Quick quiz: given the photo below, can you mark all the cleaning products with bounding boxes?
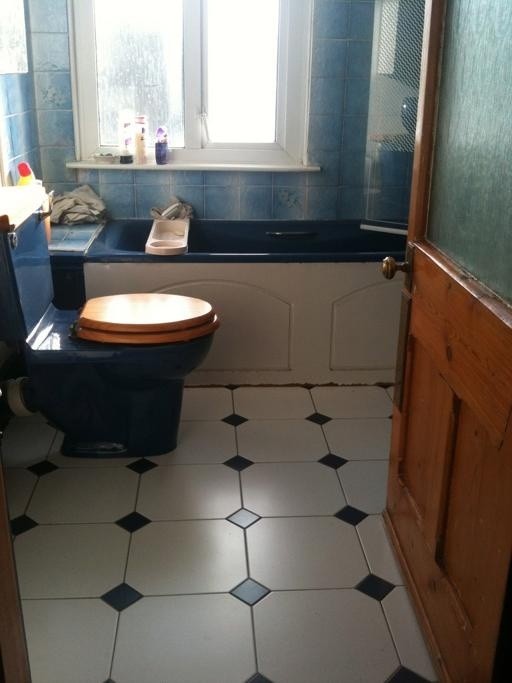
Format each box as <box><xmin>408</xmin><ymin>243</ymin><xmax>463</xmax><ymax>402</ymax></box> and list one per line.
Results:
<box><xmin>17</xmin><ymin>162</ymin><xmax>52</xmax><ymax>246</ymax></box>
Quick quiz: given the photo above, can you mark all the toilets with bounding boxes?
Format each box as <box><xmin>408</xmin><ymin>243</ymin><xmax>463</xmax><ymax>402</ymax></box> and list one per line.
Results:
<box><xmin>1</xmin><ymin>180</ymin><xmax>218</xmax><ymax>458</ymax></box>
